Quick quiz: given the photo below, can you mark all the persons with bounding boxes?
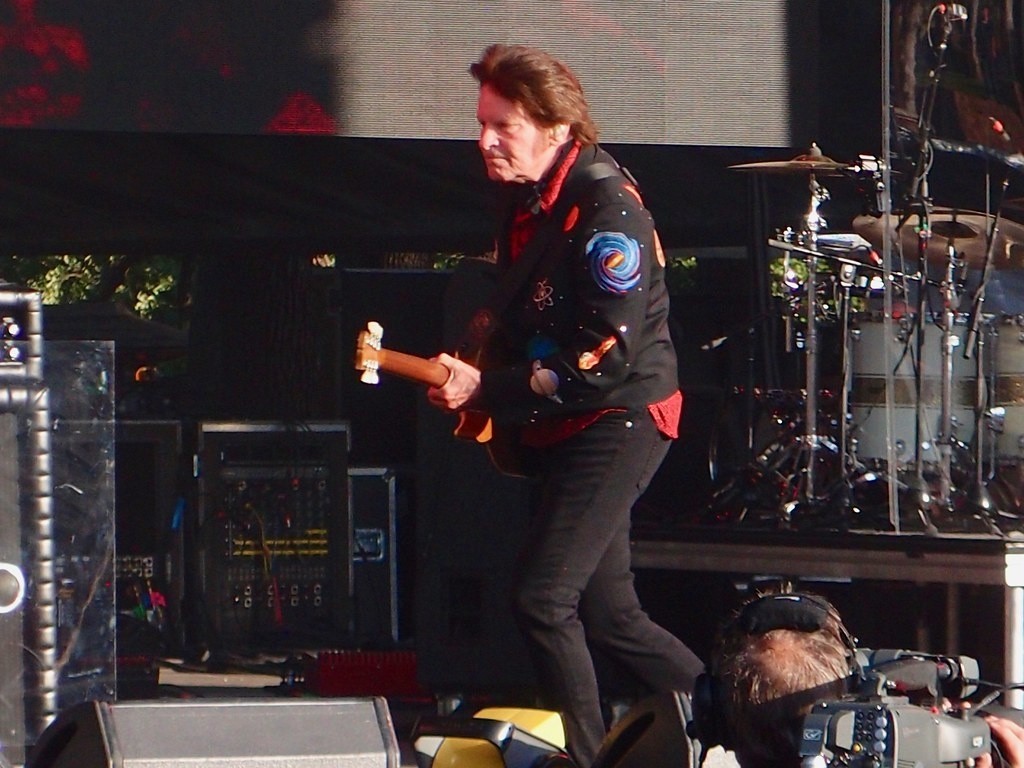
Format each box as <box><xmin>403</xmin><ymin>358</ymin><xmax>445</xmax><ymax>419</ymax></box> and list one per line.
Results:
<box><xmin>710</xmin><ymin>581</ymin><xmax>1024</xmax><ymax>768</ymax></box>
<box><xmin>427</xmin><ymin>43</ymin><xmax>708</xmax><ymax>768</ymax></box>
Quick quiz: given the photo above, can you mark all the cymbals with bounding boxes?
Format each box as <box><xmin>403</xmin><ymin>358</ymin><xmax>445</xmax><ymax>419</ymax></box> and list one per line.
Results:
<box><xmin>732</xmin><ymin>141</ymin><xmax>857</xmax><ymax>171</ymax></box>
<box><xmin>853</xmin><ymin>206</ymin><xmax>1024</xmax><ymax>270</ymax></box>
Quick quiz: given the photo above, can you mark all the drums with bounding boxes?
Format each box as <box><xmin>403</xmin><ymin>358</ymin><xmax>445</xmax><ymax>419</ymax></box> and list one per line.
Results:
<box><xmin>845</xmin><ymin>255</ymin><xmax>1024</xmax><ymax>475</ymax></box>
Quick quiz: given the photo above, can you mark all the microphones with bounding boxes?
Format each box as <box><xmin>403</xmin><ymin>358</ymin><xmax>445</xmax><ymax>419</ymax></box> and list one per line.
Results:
<box><xmin>937</xmin><ymin>4</ymin><xmax>967</xmax><ymax>19</ymax></box>
<box><xmin>989</xmin><ymin>115</ymin><xmax>1011</xmax><ymax>141</ymax></box>
<box><xmin>865</xmin><ymin>246</ymin><xmax>903</xmax><ymax>296</ymax></box>
<box><xmin>842</xmin><ymin>167</ymin><xmax>897</xmax><ymax>188</ymax></box>
<box><xmin>936</xmin><ymin>656</ymin><xmax>979</xmax><ymax>698</ymax></box>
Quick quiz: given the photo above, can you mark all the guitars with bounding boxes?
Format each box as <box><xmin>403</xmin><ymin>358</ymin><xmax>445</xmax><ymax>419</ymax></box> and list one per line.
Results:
<box><xmin>354</xmin><ymin>307</ymin><xmax>535</xmax><ymax>479</ymax></box>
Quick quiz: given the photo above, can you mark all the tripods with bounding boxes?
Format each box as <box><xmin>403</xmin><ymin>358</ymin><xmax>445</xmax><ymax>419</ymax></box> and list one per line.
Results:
<box><xmin>689</xmin><ymin>25</ymin><xmax>1024</xmax><ymax>541</ymax></box>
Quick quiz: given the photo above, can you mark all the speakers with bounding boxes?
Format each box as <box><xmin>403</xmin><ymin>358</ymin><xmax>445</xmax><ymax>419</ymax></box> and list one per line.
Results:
<box><xmin>25</xmin><ymin>695</ymin><xmax>400</xmax><ymax>768</ymax></box>
<box><xmin>337</xmin><ymin>266</ymin><xmax>559</xmax><ymax>697</ymax></box>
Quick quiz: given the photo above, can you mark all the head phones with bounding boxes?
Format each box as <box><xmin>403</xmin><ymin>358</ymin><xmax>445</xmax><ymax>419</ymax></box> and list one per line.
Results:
<box><xmin>686</xmin><ymin>594</ymin><xmax>871</xmax><ymax>750</ymax></box>
